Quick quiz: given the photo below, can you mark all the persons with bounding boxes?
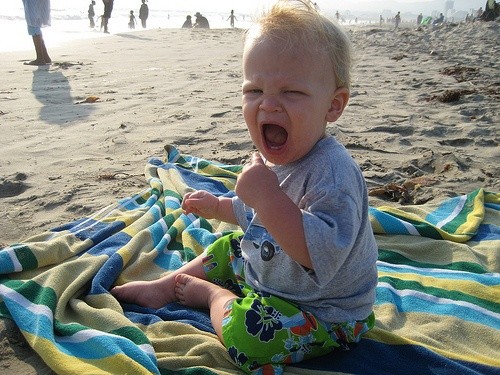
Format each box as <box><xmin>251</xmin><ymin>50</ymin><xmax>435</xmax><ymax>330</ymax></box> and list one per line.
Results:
<box><xmin>22</xmin><ymin>0</ymin><xmax>51</xmax><ymax>66</ymax></box>
<box><xmin>89</xmin><ymin>0</ymin><xmax>500</xmax><ymax>33</ymax></box>
<box><xmin>110</xmin><ymin>0</ymin><xmax>379</xmax><ymax>375</ymax></box>
